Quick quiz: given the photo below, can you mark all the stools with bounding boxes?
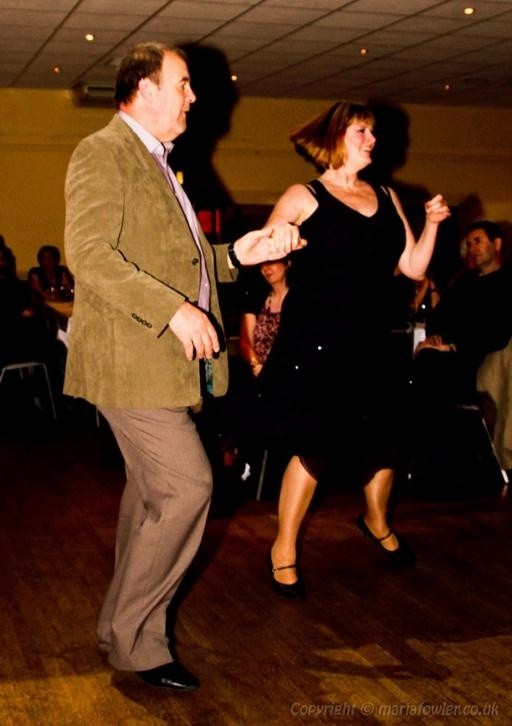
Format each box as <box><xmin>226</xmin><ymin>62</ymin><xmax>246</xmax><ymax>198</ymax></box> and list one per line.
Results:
<box><xmin>0</xmin><ymin>360</ymin><xmax>57</xmax><ymax>420</ymax></box>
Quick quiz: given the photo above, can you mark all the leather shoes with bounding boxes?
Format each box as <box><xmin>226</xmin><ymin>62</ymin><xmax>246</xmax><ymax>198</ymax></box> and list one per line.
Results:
<box><xmin>265</xmin><ymin>549</ymin><xmax>303</xmax><ymax>599</ymax></box>
<box><xmin>138</xmin><ymin>663</ymin><xmax>199</xmax><ymax>692</ymax></box>
<box><xmin>358</xmin><ymin>518</ymin><xmax>409</xmax><ymax>562</ymax></box>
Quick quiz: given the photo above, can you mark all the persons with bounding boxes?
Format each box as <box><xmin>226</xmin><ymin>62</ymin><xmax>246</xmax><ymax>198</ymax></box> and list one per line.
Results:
<box><xmin>402</xmin><ymin>220</ymin><xmax>512</xmax><ymax>405</ymax></box>
<box><xmin>244</xmin><ymin>255</ymin><xmax>290</xmax><ymax>376</ymax></box>
<box><xmin>62</xmin><ymin>41</ymin><xmax>291</xmax><ymax>694</ymax></box>
<box><xmin>26</xmin><ymin>244</ymin><xmax>73</xmax><ymax>301</ymax></box>
<box><xmin>0</xmin><ymin>235</ymin><xmax>66</xmax><ymax>396</ymax></box>
<box><xmin>261</xmin><ymin>98</ymin><xmax>451</xmax><ymax>601</ymax></box>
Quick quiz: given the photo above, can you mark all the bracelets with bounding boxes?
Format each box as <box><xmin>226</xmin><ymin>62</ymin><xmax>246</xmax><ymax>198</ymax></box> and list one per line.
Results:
<box><xmin>228</xmin><ymin>242</ymin><xmax>241</xmax><ymax>269</ymax></box>
